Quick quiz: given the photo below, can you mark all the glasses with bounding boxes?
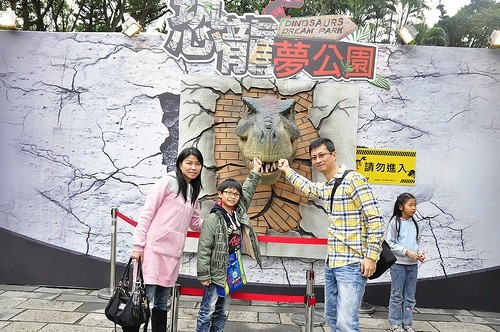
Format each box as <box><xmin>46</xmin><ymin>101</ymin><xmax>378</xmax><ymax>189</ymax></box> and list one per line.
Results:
<box><xmin>310</xmin><ymin>152</ymin><xmax>332</xmax><ymax>162</ymax></box>
<box><xmin>223</xmin><ymin>191</ymin><xmax>241</xmax><ymax>197</ymax></box>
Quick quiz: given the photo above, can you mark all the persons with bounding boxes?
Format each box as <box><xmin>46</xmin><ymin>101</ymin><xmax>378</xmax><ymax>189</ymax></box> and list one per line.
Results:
<box><xmin>278</xmin><ymin>138</ymin><xmax>384</xmax><ymax>332</ymax></box>
<box><xmin>125</xmin><ymin>148</ymin><xmax>204</xmax><ymax>332</ymax></box>
<box><xmin>387</xmin><ymin>193</ymin><xmax>426</xmax><ymax>332</ymax></box>
<box><xmin>195</xmin><ymin>156</ymin><xmax>262</xmax><ymax>332</ymax></box>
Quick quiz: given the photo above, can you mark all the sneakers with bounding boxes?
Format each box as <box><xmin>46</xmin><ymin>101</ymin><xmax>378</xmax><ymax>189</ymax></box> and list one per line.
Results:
<box><xmin>390</xmin><ymin>325</ymin><xmax>415</xmax><ymax>332</ymax></box>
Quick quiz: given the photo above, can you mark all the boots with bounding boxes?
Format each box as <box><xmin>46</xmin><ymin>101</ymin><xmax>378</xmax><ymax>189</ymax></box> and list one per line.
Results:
<box><xmin>151</xmin><ymin>307</ymin><xmax>167</xmax><ymax>332</ymax></box>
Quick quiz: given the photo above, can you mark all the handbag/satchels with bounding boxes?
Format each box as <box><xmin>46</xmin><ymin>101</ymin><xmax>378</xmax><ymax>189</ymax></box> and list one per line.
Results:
<box><xmin>105</xmin><ymin>256</ymin><xmax>150</xmax><ymax>327</ymax></box>
<box><xmin>216</xmin><ymin>249</ymin><xmax>247</xmax><ymax>297</ymax></box>
<box><xmin>367</xmin><ymin>240</ymin><xmax>397</xmax><ymax>280</ymax></box>
